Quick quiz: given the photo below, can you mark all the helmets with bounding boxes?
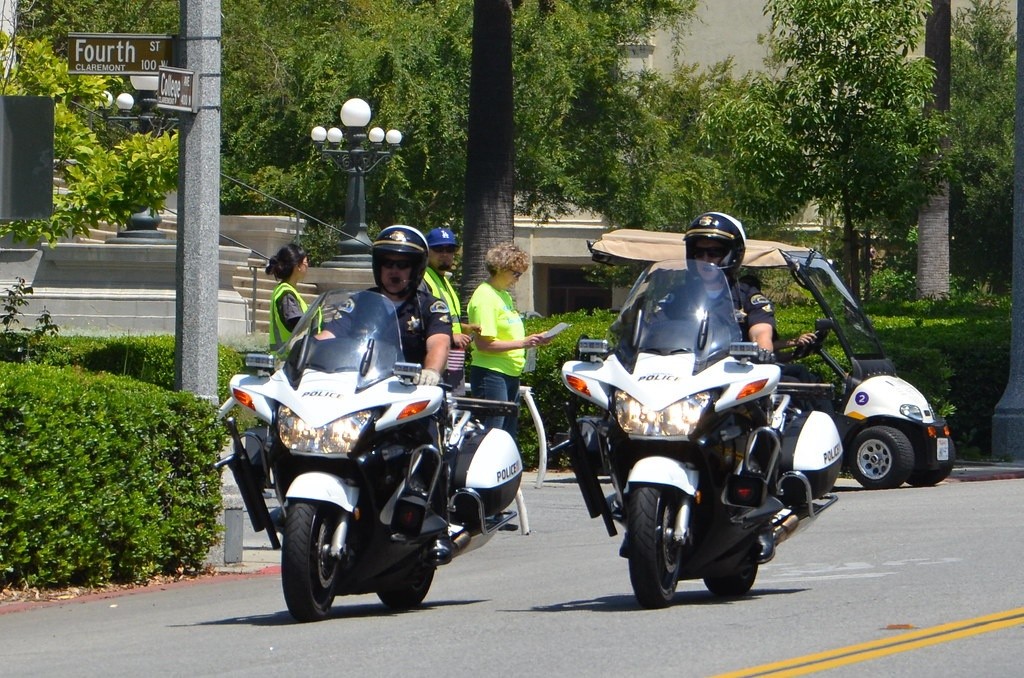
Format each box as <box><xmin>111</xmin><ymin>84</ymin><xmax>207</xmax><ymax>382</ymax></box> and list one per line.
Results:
<box><xmin>683</xmin><ymin>211</ymin><xmax>746</xmax><ymax>275</ymax></box>
<box><xmin>372</xmin><ymin>224</ymin><xmax>429</xmax><ymax>289</ymax></box>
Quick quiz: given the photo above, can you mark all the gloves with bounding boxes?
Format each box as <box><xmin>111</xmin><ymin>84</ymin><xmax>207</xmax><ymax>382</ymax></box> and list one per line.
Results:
<box><xmin>413</xmin><ymin>368</ymin><xmax>443</xmax><ymax>386</ymax></box>
<box><xmin>747</xmin><ymin>348</ymin><xmax>776</xmax><ymax>364</ymax></box>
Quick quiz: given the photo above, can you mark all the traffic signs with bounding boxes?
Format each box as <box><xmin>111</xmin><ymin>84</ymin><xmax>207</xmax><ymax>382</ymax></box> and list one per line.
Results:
<box><xmin>67</xmin><ymin>31</ymin><xmax>170</xmax><ymax>75</ymax></box>
<box><xmin>155</xmin><ymin>67</ymin><xmax>199</xmax><ymax>114</ymax></box>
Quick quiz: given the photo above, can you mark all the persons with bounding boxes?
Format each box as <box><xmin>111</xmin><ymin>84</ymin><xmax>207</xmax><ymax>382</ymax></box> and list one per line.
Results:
<box><xmin>467</xmin><ymin>241</ymin><xmax>555</xmax><ymax>531</ymax></box>
<box><xmin>650</xmin><ymin>212</ymin><xmax>772</xmax><ymax>471</ymax></box>
<box><xmin>316</xmin><ymin>225</ymin><xmax>453</xmax><ymax>564</ymax></box>
<box><xmin>738</xmin><ymin>275</ymin><xmax>836</xmax><ymax>419</ymax></box>
<box><xmin>417</xmin><ymin>227</ymin><xmax>481</xmax><ymax>398</ymax></box>
<box><xmin>265</xmin><ymin>243</ymin><xmax>308</xmax><ymax>360</ymax></box>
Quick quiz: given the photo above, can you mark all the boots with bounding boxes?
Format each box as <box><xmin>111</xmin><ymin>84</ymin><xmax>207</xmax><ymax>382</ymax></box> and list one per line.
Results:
<box><xmin>269</xmin><ymin>506</ymin><xmax>290</xmax><ymax>529</ymax></box>
<box><xmin>606</xmin><ymin>492</ymin><xmax>630</xmax><ymax>520</ymax></box>
<box><xmin>741</xmin><ymin>429</ymin><xmax>783</xmax><ymax>564</ymax></box>
<box><xmin>425</xmin><ymin>461</ymin><xmax>452</xmax><ymax>565</ymax></box>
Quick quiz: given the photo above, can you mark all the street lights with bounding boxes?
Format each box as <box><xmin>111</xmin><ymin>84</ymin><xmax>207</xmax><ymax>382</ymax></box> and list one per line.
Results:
<box><xmin>93</xmin><ymin>75</ymin><xmax>179</xmax><ymax>246</ymax></box>
<box><xmin>310</xmin><ymin>97</ymin><xmax>403</xmax><ymax>266</ymax></box>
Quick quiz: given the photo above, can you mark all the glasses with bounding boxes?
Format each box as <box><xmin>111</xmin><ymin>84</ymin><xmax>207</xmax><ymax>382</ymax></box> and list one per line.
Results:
<box><xmin>509</xmin><ymin>269</ymin><xmax>522</xmax><ymax>278</ymax></box>
<box><xmin>381</xmin><ymin>258</ymin><xmax>414</xmax><ymax>270</ymax></box>
<box><xmin>431</xmin><ymin>245</ymin><xmax>455</xmax><ymax>253</ymax></box>
<box><xmin>694</xmin><ymin>246</ymin><xmax>726</xmax><ymax>257</ymax></box>
<box><xmin>301</xmin><ymin>262</ymin><xmax>308</xmax><ymax>269</ymax></box>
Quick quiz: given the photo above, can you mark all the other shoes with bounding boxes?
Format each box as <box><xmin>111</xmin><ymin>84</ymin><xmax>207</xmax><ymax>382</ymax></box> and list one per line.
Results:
<box><xmin>495</xmin><ymin>514</ymin><xmax>518</xmax><ymax>531</ymax></box>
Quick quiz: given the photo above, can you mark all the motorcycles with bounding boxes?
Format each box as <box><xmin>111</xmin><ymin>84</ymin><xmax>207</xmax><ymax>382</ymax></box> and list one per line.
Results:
<box><xmin>554</xmin><ymin>260</ymin><xmax>844</xmax><ymax>610</ymax></box>
<box><xmin>216</xmin><ymin>289</ymin><xmax>525</xmax><ymax>621</ymax></box>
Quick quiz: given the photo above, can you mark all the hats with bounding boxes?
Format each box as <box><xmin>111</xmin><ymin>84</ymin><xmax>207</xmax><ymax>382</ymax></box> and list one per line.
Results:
<box><xmin>425</xmin><ymin>227</ymin><xmax>457</xmax><ymax>247</ymax></box>
